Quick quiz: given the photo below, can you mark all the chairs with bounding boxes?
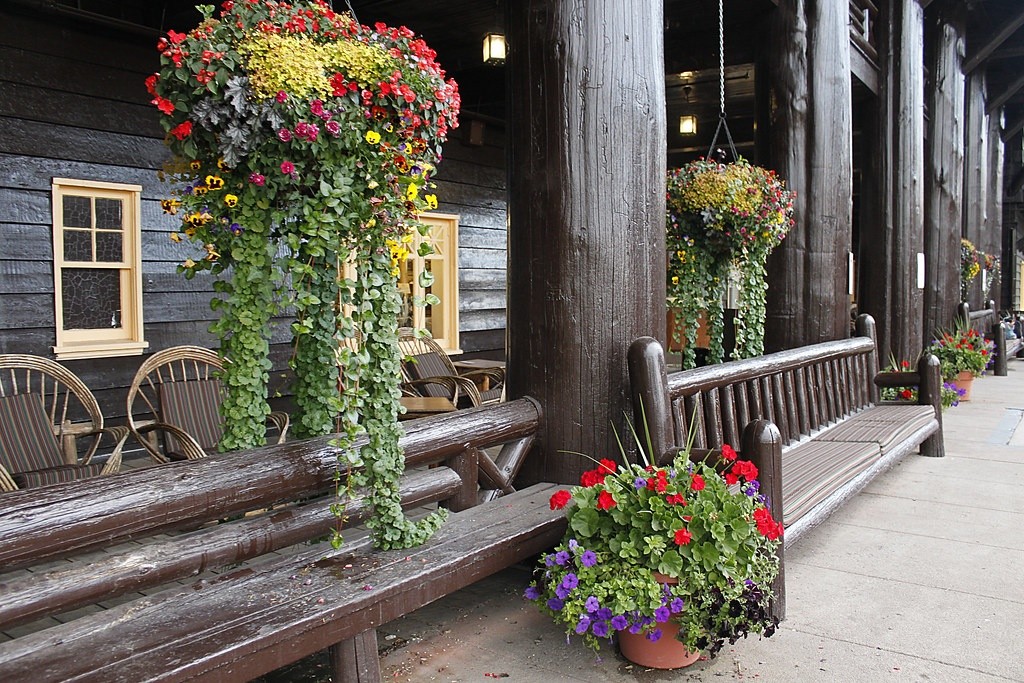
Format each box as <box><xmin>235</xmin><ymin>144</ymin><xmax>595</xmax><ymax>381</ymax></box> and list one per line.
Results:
<box><xmin>0</xmin><ymin>353</ymin><xmax>131</xmax><ymax>494</ymax></box>
<box><xmin>127</xmin><ymin>345</ymin><xmax>302</xmax><ymax>526</ymax></box>
<box><xmin>396</xmin><ymin>327</ymin><xmax>507</xmax><ymax>407</ymax></box>
<box><xmin>337</xmin><ymin>324</ymin><xmax>460</xmax><ymax>423</ymax></box>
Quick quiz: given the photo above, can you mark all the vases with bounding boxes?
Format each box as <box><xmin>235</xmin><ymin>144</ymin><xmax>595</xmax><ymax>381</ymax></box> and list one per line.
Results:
<box><xmin>615</xmin><ymin>571</ymin><xmax>701</xmax><ymax>669</ymax></box>
<box><xmin>946</xmin><ymin>369</ymin><xmax>974</xmax><ymax>402</ymax></box>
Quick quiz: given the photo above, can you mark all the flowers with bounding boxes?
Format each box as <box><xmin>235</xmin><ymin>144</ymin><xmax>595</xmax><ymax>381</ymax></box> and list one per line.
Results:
<box><xmin>667</xmin><ymin>148</ymin><xmax>800</xmax><ymax>381</ymax></box>
<box><xmin>143</xmin><ymin>0</ymin><xmax>452</xmax><ymax>558</ymax></box>
<box><xmin>879</xmin><ymin>347</ymin><xmax>966</xmax><ymax>412</ymax></box>
<box><xmin>982</xmin><ymin>253</ymin><xmax>1003</xmax><ymax>296</ymax></box>
<box><xmin>960</xmin><ymin>238</ymin><xmax>984</xmax><ymax>300</ymax></box>
<box><xmin>929</xmin><ymin>316</ymin><xmax>998</xmax><ymax>380</ymax></box>
<box><xmin>519</xmin><ymin>392</ymin><xmax>784</xmax><ymax>660</ymax></box>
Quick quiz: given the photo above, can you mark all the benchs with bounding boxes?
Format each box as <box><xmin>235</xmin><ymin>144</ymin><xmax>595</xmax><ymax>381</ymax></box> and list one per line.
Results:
<box><xmin>958</xmin><ymin>300</ymin><xmax>1024</xmax><ymax>377</ymax></box>
<box><xmin>0</xmin><ymin>394</ymin><xmax>585</xmax><ymax>683</ymax></box>
<box><xmin>625</xmin><ymin>313</ymin><xmax>947</xmax><ymax>623</ymax></box>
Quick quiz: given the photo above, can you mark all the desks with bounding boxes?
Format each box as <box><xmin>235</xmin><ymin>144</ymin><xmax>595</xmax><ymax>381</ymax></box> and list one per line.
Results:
<box><xmin>452</xmin><ymin>358</ymin><xmax>506</xmax><ymax>390</ymax></box>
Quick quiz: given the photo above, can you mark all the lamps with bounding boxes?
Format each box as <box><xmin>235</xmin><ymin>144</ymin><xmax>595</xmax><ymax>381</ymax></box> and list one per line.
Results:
<box><xmin>481</xmin><ymin>32</ymin><xmax>506</xmax><ymax>65</ymax></box>
<box><xmin>677</xmin><ymin>85</ymin><xmax>700</xmax><ymax>138</ymax></box>
